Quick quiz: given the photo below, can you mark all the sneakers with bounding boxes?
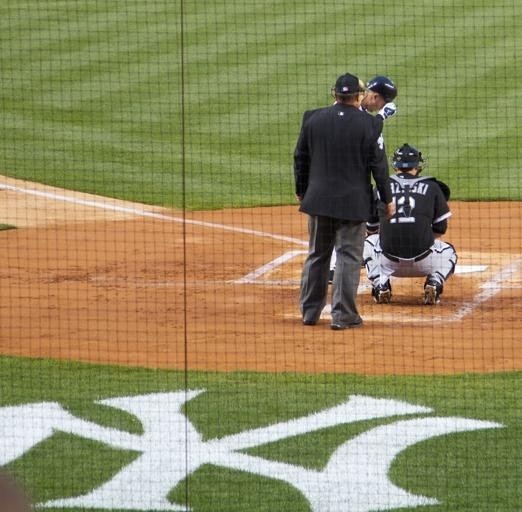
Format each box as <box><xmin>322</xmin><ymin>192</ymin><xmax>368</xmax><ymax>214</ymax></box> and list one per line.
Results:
<box><xmin>304</xmin><ymin>319</ymin><xmax>317</xmax><ymax>325</ymax></box>
<box><xmin>424</xmin><ymin>279</ymin><xmax>440</xmax><ymax>305</ymax></box>
<box><xmin>375</xmin><ymin>283</ymin><xmax>391</xmax><ymax>304</ymax></box>
<box><xmin>331</xmin><ymin>320</ymin><xmax>362</xmax><ymax>330</ymax></box>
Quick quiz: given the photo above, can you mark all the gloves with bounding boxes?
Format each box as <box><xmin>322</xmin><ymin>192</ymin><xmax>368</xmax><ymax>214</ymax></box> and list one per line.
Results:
<box><xmin>378</xmin><ymin>102</ymin><xmax>397</xmax><ymax>119</ymax></box>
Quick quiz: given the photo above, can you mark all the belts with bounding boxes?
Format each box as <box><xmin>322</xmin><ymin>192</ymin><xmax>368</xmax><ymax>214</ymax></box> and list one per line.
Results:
<box><xmin>381</xmin><ymin>251</ymin><xmax>432</xmax><ymax>262</ymax></box>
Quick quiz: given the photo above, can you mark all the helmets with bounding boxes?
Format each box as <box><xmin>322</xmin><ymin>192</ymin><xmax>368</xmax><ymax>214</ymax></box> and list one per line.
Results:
<box><xmin>392</xmin><ymin>144</ymin><xmax>423</xmax><ymax>175</ymax></box>
<box><xmin>331</xmin><ymin>73</ymin><xmax>365</xmax><ymax>96</ymax></box>
<box><xmin>366</xmin><ymin>76</ymin><xmax>397</xmax><ymax>102</ymax></box>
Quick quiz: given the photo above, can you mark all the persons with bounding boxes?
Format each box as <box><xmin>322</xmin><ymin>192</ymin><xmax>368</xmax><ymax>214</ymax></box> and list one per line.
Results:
<box><xmin>292</xmin><ymin>71</ymin><xmax>392</xmax><ymax>332</ymax></box>
<box><xmin>362</xmin><ymin>143</ymin><xmax>458</xmax><ymax>305</ymax></box>
<box><xmin>327</xmin><ymin>75</ymin><xmax>398</xmax><ymax>287</ymax></box>
